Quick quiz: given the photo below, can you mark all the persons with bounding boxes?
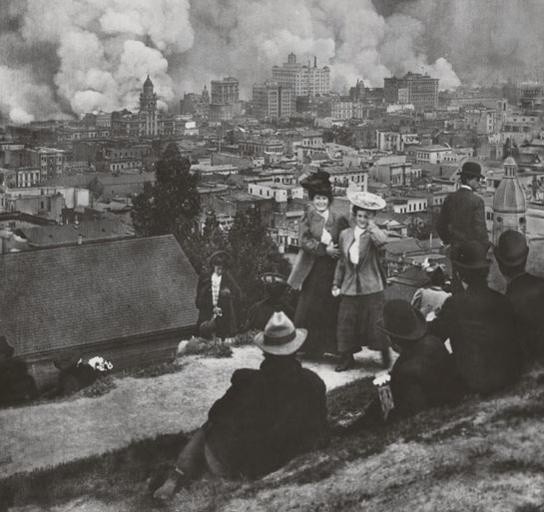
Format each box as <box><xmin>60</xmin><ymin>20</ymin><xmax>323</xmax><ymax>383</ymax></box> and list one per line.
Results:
<box><xmin>287</xmin><ymin>172</ymin><xmax>392</xmax><ymax>372</ymax></box>
<box><xmin>363</xmin><ymin>162</ymin><xmax>544</xmax><ymax>422</ymax></box>
<box><xmin>194</xmin><ymin>251</ymin><xmax>241</xmax><ymax>342</ymax></box>
<box><xmin>153</xmin><ymin>310</ymin><xmax>328</xmax><ymax>504</ymax></box>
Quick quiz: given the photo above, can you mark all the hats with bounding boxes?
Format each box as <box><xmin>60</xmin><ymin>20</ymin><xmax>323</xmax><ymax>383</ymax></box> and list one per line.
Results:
<box><xmin>376</xmin><ymin>299</ymin><xmax>428</xmax><ymax>341</ymax></box>
<box><xmin>495</xmin><ymin>229</ymin><xmax>526</xmax><ymax>268</ymax></box>
<box><xmin>346</xmin><ymin>191</ymin><xmax>386</xmax><ymax>211</ymax></box>
<box><xmin>443</xmin><ymin>236</ymin><xmax>494</xmax><ymax>272</ymax></box>
<box><xmin>300</xmin><ymin>166</ymin><xmax>331</xmax><ymax>189</ymax></box>
<box><xmin>253</xmin><ymin>311</ymin><xmax>308</xmax><ymax>356</ymax></box>
<box><xmin>209</xmin><ymin>250</ymin><xmax>230</xmax><ymax>268</ymax></box>
<box><xmin>457</xmin><ymin>162</ymin><xmax>484</xmax><ymax>178</ymax></box>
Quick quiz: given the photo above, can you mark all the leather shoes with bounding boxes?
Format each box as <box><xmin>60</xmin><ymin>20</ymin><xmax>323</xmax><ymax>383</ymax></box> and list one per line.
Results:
<box><xmin>335</xmin><ymin>356</ymin><xmax>355</xmax><ymax>372</ymax></box>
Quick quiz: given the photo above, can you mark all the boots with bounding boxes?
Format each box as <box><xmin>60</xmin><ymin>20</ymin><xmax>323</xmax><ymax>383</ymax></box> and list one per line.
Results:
<box><xmin>151</xmin><ymin>469</ymin><xmax>183</xmax><ymax>505</ymax></box>
